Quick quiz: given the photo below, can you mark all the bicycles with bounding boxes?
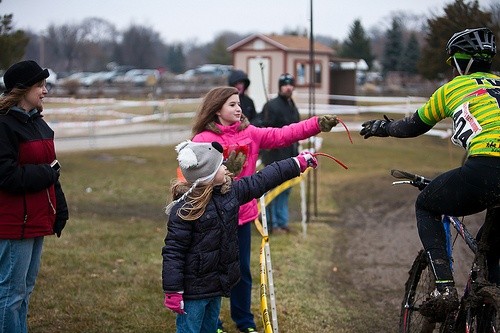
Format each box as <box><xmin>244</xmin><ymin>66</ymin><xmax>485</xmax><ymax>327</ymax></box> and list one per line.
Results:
<box><xmin>390</xmin><ymin>169</ymin><xmax>500</xmax><ymax>333</ymax></box>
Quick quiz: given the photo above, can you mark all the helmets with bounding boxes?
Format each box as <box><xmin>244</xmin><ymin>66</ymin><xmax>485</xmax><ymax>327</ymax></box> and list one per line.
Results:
<box><xmin>445</xmin><ymin>28</ymin><xmax>497</xmax><ymax>71</ymax></box>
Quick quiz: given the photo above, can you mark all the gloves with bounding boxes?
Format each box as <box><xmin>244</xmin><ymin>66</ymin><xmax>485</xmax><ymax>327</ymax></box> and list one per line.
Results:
<box><xmin>164</xmin><ymin>293</ymin><xmax>186</xmax><ymax>315</ymax></box>
<box><xmin>54</xmin><ymin>219</ymin><xmax>67</xmax><ymax>238</ymax></box>
<box><xmin>359</xmin><ymin>114</ymin><xmax>394</xmax><ymax>139</ymax></box>
<box><xmin>50</xmin><ymin>164</ymin><xmax>61</xmax><ymax>184</ymax></box>
<box><xmin>318</xmin><ymin>115</ymin><xmax>340</xmax><ymax>133</ymax></box>
<box><xmin>296</xmin><ymin>152</ymin><xmax>318</xmax><ymax>174</ymax></box>
<box><xmin>223</xmin><ymin>149</ymin><xmax>247</xmax><ymax>177</ymax></box>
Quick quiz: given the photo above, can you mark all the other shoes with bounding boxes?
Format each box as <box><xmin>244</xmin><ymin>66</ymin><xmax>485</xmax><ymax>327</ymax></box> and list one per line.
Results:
<box><xmin>216</xmin><ymin>327</ymin><xmax>228</xmax><ymax>333</ymax></box>
<box><xmin>419</xmin><ymin>280</ymin><xmax>458</xmax><ymax>316</ymax></box>
<box><xmin>280</xmin><ymin>224</ymin><xmax>298</xmax><ymax>235</ymax></box>
<box><xmin>240</xmin><ymin>328</ymin><xmax>258</xmax><ymax>333</ymax></box>
<box><xmin>268</xmin><ymin>227</ymin><xmax>286</xmax><ymax>236</ymax></box>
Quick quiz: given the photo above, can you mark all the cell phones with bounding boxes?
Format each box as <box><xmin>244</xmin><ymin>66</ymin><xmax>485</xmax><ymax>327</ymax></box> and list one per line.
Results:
<box><xmin>50</xmin><ymin>159</ymin><xmax>61</xmax><ymax>171</ymax></box>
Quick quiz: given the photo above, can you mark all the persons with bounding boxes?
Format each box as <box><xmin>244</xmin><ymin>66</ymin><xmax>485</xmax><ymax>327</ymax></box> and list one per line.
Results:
<box><xmin>225</xmin><ymin>65</ymin><xmax>261</xmax><ymax>129</ymax></box>
<box><xmin>360</xmin><ymin>27</ymin><xmax>499</xmax><ymax>333</ymax></box>
<box><xmin>158</xmin><ymin>140</ymin><xmax>319</xmax><ymax>333</ymax></box>
<box><xmin>170</xmin><ymin>85</ymin><xmax>342</xmax><ymax>333</ymax></box>
<box><xmin>256</xmin><ymin>73</ymin><xmax>302</xmax><ymax>232</ymax></box>
<box><xmin>0</xmin><ymin>57</ymin><xmax>69</xmax><ymax>333</ymax></box>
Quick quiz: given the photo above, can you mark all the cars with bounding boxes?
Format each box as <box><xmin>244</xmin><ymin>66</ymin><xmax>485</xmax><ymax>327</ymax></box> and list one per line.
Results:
<box><xmin>0</xmin><ymin>64</ymin><xmax>232</xmax><ymax>100</ymax></box>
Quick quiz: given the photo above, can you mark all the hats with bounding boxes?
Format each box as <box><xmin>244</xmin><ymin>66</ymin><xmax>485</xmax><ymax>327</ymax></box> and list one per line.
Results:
<box><xmin>278</xmin><ymin>72</ymin><xmax>294</xmax><ymax>88</ymax></box>
<box><xmin>164</xmin><ymin>139</ymin><xmax>225</xmax><ymax>215</ymax></box>
<box><xmin>3</xmin><ymin>60</ymin><xmax>50</xmax><ymax>94</ymax></box>
